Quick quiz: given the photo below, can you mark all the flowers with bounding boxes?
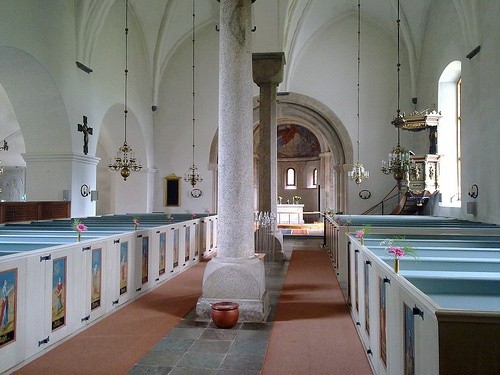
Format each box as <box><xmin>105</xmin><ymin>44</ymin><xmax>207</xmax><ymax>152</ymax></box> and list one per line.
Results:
<box><xmin>380</xmin><ymin>236</ymin><xmax>417</xmax><ymax>260</ymax></box>
<box><xmin>72</xmin><ymin>218</ymin><xmax>88</xmax><ymax>232</ymax></box>
<box><xmin>295</xmin><ymin>195</ymin><xmax>301</xmax><ymax>201</ymax></box>
<box><xmin>355</xmin><ymin>224</ymin><xmax>372</xmax><ymax>237</ymax></box>
<box><xmin>133</xmin><ymin>218</ymin><xmax>141</xmax><ymax>225</ymax></box>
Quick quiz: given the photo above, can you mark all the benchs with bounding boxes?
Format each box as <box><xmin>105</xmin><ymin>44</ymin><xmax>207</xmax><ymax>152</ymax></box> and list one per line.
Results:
<box><xmin>323</xmin><ymin>212</ymin><xmax>500</xmax><ymax>375</ymax></box>
<box><xmin>0</xmin><ymin>214</ymin><xmax>217</xmax><ymax>375</ymax></box>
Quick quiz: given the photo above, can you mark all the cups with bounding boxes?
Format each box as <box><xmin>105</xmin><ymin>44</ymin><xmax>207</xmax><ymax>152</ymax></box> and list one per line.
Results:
<box><xmin>63</xmin><ymin>190</ymin><xmax>70</xmax><ymax>201</ymax></box>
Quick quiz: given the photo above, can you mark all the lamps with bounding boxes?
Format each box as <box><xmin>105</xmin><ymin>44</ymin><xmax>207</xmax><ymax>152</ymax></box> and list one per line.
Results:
<box><xmin>183</xmin><ymin>0</ymin><xmax>203</xmax><ymax>188</ymax></box>
<box><xmin>381</xmin><ymin>0</ymin><xmax>418</xmax><ymax>189</ymax></box>
<box><xmin>87</xmin><ymin>189</ymin><xmax>98</xmax><ymax>201</ymax></box>
<box><xmin>108</xmin><ymin>0</ymin><xmax>142</xmax><ymax>181</ymax></box>
<box><xmin>467</xmin><ymin>202</ymin><xmax>476</xmax><ymax>215</ymax></box>
<box><xmin>348</xmin><ymin>0</ymin><xmax>369</xmax><ymax>185</ymax></box>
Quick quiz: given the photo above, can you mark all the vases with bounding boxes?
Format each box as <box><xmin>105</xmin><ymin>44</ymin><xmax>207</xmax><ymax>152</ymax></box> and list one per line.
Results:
<box><xmin>78</xmin><ymin>232</ymin><xmax>80</xmax><ymax>242</ymax></box>
<box><xmin>361</xmin><ymin>237</ymin><xmax>364</xmax><ymax>245</ymax></box>
<box><xmin>135</xmin><ymin>224</ymin><xmax>138</xmax><ymax>231</ymax></box>
<box><xmin>212</xmin><ymin>302</ymin><xmax>239</xmax><ymax>329</ymax></box>
<box><xmin>394</xmin><ymin>258</ymin><xmax>399</xmax><ymax>273</ymax></box>
<box><xmin>296</xmin><ymin>201</ymin><xmax>299</xmax><ymax>204</ymax></box>
